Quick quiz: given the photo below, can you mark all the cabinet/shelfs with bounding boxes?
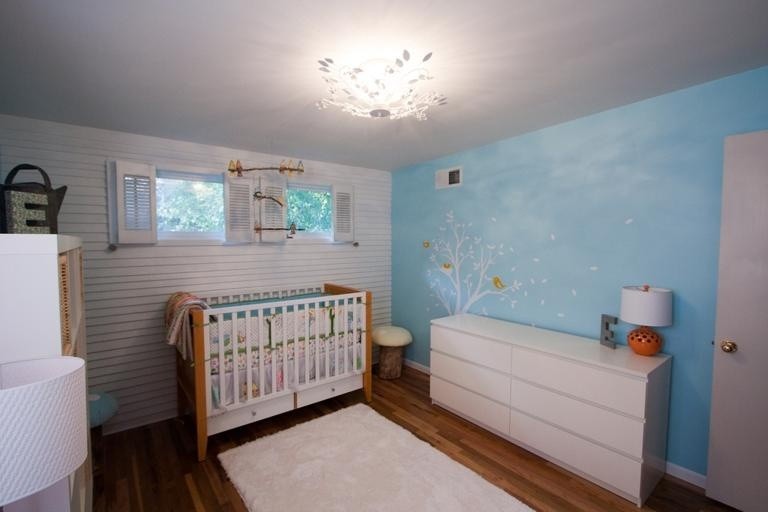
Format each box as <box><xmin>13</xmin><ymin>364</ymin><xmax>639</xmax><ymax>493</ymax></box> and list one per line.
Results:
<box><xmin>429</xmin><ymin>313</ymin><xmax>672</xmax><ymax>509</ymax></box>
<box><xmin>0</xmin><ymin>234</ymin><xmax>96</xmax><ymax>512</ymax></box>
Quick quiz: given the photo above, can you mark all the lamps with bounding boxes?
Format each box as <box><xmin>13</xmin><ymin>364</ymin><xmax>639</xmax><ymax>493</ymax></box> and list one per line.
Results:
<box><xmin>619</xmin><ymin>283</ymin><xmax>673</xmax><ymax>356</ymax></box>
<box><xmin>314</xmin><ymin>45</ymin><xmax>448</xmax><ymax>124</ymax></box>
<box><xmin>0</xmin><ymin>356</ymin><xmax>88</xmax><ymax>512</ymax></box>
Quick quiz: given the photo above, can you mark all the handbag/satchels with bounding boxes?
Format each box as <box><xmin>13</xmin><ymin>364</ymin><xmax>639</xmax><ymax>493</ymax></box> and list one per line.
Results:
<box><xmin>1</xmin><ymin>164</ymin><xmax>67</xmax><ymax>234</ymax></box>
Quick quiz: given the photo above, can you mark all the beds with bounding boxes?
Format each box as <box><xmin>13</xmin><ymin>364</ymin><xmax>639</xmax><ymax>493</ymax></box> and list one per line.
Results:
<box><xmin>176</xmin><ymin>283</ymin><xmax>373</xmax><ymax>464</ymax></box>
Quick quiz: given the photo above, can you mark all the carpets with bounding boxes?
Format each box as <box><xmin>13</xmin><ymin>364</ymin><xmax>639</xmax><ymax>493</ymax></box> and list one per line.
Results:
<box><xmin>217</xmin><ymin>403</ymin><xmax>536</xmax><ymax>512</ymax></box>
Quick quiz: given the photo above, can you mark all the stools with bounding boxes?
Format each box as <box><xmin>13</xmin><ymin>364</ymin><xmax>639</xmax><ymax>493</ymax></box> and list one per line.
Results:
<box><xmin>373</xmin><ymin>326</ymin><xmax>413</xmax><ymax>380</ymax></box>
<box><xmin>87</xmin><ymin>389</ymin><xmax>118</xmax><ymax>466</ymax></box>
